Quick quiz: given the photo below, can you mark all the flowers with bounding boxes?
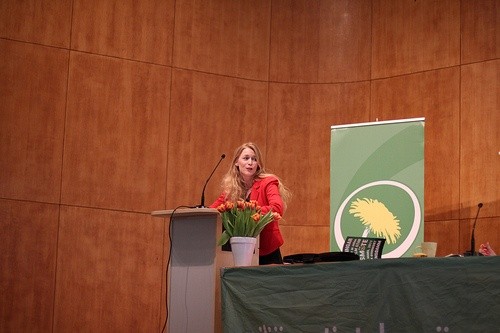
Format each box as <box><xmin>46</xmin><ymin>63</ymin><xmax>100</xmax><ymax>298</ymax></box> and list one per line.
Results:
<box><xmin>217</xmin><ymin>201</ymin><xmax>282</xmax><ymax>245</ymax></box>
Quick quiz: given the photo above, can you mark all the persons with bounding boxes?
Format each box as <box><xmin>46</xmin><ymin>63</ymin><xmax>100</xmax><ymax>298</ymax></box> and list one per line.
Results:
<box><xmin>207</xmin><ymin>142</ymin><xmax>290</xmax><ymax>265</ymax></box>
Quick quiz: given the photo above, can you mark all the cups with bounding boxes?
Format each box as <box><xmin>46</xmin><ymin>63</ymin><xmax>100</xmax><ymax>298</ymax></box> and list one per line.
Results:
<box><xmin>415</xmin><ymin>242</ymin><xmax>437</xmax><ymax>258</ymax></box>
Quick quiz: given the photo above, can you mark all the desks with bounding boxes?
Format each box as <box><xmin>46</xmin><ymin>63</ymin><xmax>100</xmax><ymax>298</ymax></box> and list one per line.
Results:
<box><xmin>220</xmin><ymin>256</ymin><xmax>500</xmax><ymax>333</ymax></box>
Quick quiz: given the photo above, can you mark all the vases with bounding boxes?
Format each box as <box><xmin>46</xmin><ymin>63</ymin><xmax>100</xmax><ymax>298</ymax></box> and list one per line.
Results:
<box><xmin>230</xmin><ymin>237</ymin><xmax>256</xmax><ymax>266</ymax></box>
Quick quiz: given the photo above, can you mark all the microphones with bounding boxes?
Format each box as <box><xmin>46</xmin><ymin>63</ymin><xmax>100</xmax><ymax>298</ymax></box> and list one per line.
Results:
<box><xmin>199</xmin><ymin>154</ymin><xmax>226</xmax><ymax>208</ymax></box>
<box><xmin>471</xmin><ymin>202</ymin><xmax>483</xmax><ymax>251</ymax></box>
